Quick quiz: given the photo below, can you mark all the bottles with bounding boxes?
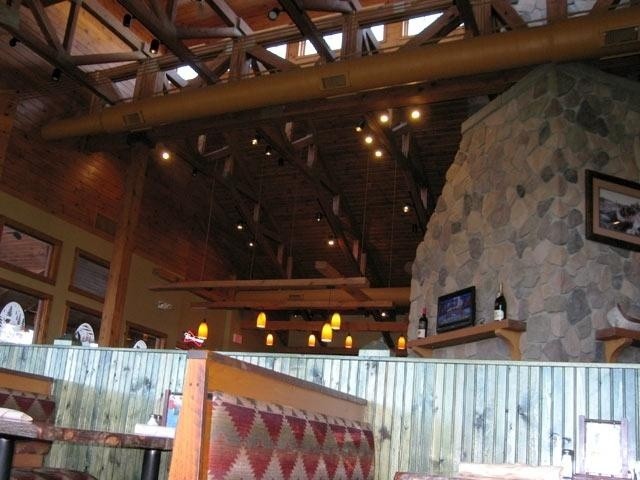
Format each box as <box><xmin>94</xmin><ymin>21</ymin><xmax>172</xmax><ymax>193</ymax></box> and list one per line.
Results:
<box><xmin>418</xmin><ymin>305</ymin><xmax>427</xmax><ymax>338</ymax></box>
<box><xmin>148</xmin><ymin>412</ymin><xmax>162</xmax><ymax>427</ymax></box>
<box><xmin>561</xmin><ymin>439</ymin><xmax>574</xmax><ymax>478</ymax></box>
<box><xmin>494</xmin><ymin>281</ymin><xmax>506</xmax><ymax>322</ymax></box>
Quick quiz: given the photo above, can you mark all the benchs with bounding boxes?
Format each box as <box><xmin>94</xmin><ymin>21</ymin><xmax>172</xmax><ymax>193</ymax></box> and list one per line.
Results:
<box><xmin>0</xmin><ymin>367</ymin><xmax>100</xmax><ymax>480</ymax></box>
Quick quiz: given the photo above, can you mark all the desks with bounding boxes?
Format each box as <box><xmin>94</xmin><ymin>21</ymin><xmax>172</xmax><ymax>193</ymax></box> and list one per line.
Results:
<box><xmin>0</xmin><ymin>414</ymin><xmax>173</xmax><ymax>480</ymax></box>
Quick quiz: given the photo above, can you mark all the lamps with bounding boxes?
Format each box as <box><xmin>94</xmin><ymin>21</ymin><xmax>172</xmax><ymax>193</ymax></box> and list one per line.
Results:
<box><xmin>197</xmin><ymin>303</ymin><xmax>407</xmax><ymax>352</ymax></box>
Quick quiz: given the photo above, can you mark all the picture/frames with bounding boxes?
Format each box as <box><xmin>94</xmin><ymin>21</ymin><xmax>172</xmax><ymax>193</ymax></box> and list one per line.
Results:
<box><xmin>582</xmin><ymin>168</ymin><xmax>638</xmax><ymax>255</ymax></box>
